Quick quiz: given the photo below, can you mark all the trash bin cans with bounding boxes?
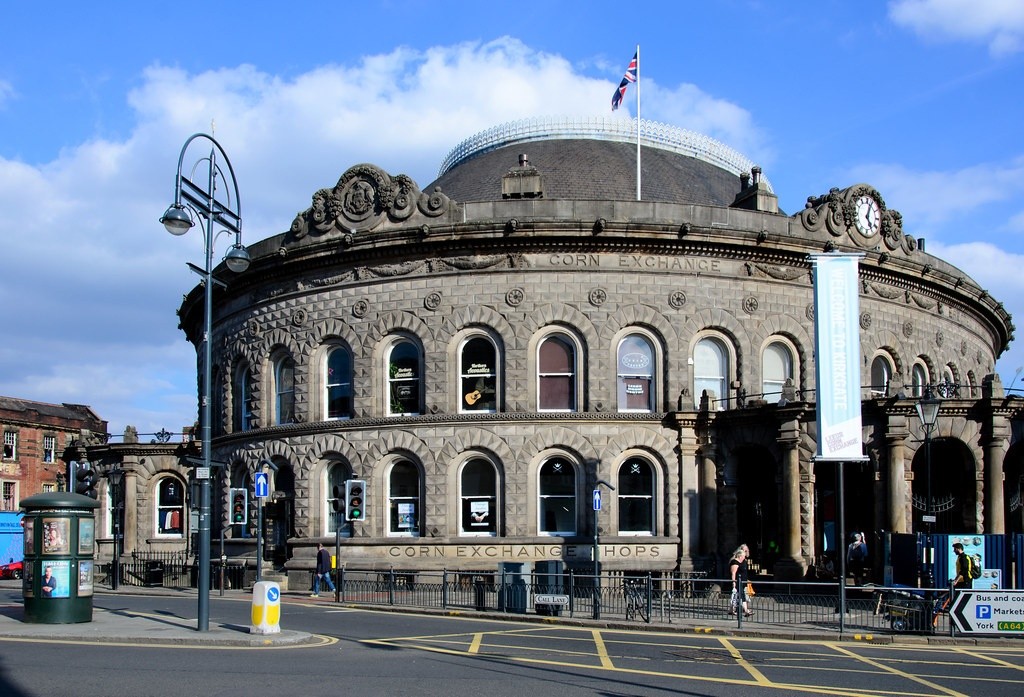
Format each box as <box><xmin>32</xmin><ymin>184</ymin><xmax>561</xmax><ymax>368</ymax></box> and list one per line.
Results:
<box><xmin>474</xmin><ymin>575</ymin><xmax>487</xmax><ymax>611</ymax></box>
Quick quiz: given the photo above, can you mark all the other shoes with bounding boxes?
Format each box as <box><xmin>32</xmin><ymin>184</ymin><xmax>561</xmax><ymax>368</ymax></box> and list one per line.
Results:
<box><xmin>332</xmin><ymin>589</ymin><xmax>336</xmax><ymax>598</ymax></box>
<box><xmin>728</xmin><ymin>608</ymin><xmax>737</xmax><ymax>615</ymax></box>
<box><xmin>743</xmin><ymin>608</ymin><xmax>755</xmax><ymax>617</ymax></box>
<box><xmin>310</xmin><ymin>594</ymin><xmax>318</xmax><ymax>598</ymax></box>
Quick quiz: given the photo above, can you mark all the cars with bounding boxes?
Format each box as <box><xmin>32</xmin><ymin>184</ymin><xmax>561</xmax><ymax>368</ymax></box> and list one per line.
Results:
<box><xmin>0</xmin><ymin>561</ymin><xmax>23</xmax><ymax>579</ymax></box>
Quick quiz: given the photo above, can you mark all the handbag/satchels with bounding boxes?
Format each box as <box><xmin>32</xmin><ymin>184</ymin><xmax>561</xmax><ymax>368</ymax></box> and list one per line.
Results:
<box><xmin>747</xmin><ymin>580</ymin><xmax>755</xmax><ymax>597</ymax></box>
<box><xmin>730</xmin><ymin>587</ymin><xmax>737</xmax><ymax>607</ymax></box>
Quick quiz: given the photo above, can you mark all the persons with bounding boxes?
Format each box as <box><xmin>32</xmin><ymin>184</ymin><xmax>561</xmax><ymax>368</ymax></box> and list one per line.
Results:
<box><xmin>310</xmin><ymin>543</ymin><xmax>337</xmax><ymax>597</ymax></box>
<box><xmin>847</xmin><ymin>532</ymin><xmax>868</xmax><ymax>586</ymax></box>
<box><xmin>41</xmin><ymin>566</ymin><xmax>56</xmax><ymax>598</ymax></box>
<box><xmin>950</xmin><ymin>543</ymin><xmax>981</xmax><ymax>589</ymax></box>
<box><xmin>728</xmin><ymin>544</ymin><xmax>756</xmax><ymax>617</ymax></box>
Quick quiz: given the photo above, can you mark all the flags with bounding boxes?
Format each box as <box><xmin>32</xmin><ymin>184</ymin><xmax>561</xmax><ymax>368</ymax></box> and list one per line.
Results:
<box><xmin>612</xmin><ymin>53</ymin><xmax>637</xmax><ymax>110</ymax></box>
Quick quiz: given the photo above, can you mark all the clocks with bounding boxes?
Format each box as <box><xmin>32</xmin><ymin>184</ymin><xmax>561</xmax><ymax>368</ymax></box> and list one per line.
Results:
<box><xmin>854</xmin><ymin>196</ymin><xmax>881</xmax><ymax>238</ymax></box>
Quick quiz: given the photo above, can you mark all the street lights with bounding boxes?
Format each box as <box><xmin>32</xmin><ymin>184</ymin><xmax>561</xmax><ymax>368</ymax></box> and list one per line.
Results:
<box><xmin>160</xmin><ymin>133</ymin><xmax>251</xmax><ymax>635</ymax></box>
<box><xmin>915</xmin><ymin>382</ymin><xmax>940</xmax><ymax>632</ymax></box>
<box><xmin>108</xmin><ymin>468</ymin><xmax>123</xmax><ymax>590</ymax></box>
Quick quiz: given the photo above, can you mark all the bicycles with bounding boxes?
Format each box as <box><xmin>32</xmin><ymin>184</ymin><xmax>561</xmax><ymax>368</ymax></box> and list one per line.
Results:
<box><xmin>619</xmin><ymin>579</ymin><xmax>649</xmax><ymax>622</ymax></box>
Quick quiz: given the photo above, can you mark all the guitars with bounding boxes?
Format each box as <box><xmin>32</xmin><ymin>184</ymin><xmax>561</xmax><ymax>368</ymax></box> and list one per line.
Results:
<box><xmin>464</xmin><ymin>387</ymin><xmax>489</xmax><ymax>405</ymax></box>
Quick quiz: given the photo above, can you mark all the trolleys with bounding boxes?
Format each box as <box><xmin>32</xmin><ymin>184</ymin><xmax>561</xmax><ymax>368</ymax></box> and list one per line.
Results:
<box><xmin>862</xmin><ymin>582</ymin><xmax>957</xmax><ymax>632</ymax></box>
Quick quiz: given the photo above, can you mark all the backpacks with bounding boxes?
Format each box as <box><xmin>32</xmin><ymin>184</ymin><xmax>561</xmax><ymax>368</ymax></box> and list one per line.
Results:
<box><xmin>960</xmin><ymin>554</ymin><xmax>981</xmax><ymax>579</ymax></box>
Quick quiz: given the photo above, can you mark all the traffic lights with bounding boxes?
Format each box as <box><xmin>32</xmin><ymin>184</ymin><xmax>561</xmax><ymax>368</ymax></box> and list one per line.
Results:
<box><xmin>76</xmin><ymin>468</ymin><xmax>98</xmax><ymax>500</ymax></box>
<box><xmin>346</xmin><ymin>479</ymin><xmax>366</xmax><ymax>522</ymax></box>
<box><xmin>333</xmin><ymin>482</ymin><xmax>346</xmax><ymax>514</ymax></box>
<box><xmin>230</xmin><ymin>488</ymin><xmax>248</xmax><ymax>525</ymax></box>
